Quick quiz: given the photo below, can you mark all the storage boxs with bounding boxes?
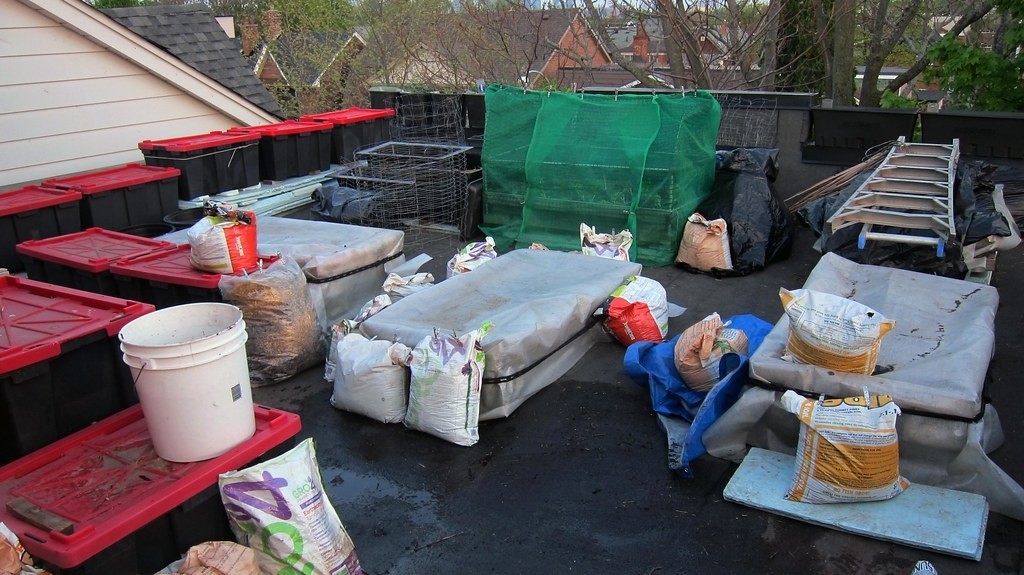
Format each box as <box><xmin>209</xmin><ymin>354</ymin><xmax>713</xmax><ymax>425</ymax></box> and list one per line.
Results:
<box><xmin>0</xmin><ymin>400</ymin><xmax>302</xmax><ymax>575</ymax></box>
<box><xmin>138</xmin><ymin>130</ymin><xmax>263</xmax><ymax>201</ymax></box>
<box><xmin>40</xmin><ymin>162</ymin><xmax>181</xmax><ymax>230</ymax></box>
<box><xmin>108</xmin><ymin>244</ymin><xmax>280</xmax><ymax>311</ymax></box>
<box><xmin>15</xmin><ymin>227</ymin><xmax>178</xmax><ymax>295</ymax></box>
<box><xmin>0</xmin><ymin>276</ymin><xmax>156</xmax><ymax>467</ymax></box>
<box><xmin>228</xmin><ymin>119</ymin><xmax>334</xmax><ymax>182</ymax></box>
<box><xmin>300</xmin><ymin>107</ymin><xmax>396</xmax><ymax>165</ymax></box>
<box><xmin>1</xmin><ymin>183</ymin><xmax>83</xmax><ymax>276</ymax></box>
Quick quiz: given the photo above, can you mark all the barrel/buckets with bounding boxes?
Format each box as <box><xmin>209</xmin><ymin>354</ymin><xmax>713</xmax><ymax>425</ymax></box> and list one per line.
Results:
<box><xmin>118</xmin><ymin>302</ymin><xmax>256</xmax><ymax>462</ymax></box>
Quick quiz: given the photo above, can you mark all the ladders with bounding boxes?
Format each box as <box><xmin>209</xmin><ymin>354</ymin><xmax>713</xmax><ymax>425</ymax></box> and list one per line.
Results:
<box><xmin>857</xmin><ymin>191</ymin><xmax>946</xmax><ymax>258</ymax></box>
<box><xmin>823</xmin><ymin>136</ymin><xmax>960</xmax><ymax>249</ymax></box>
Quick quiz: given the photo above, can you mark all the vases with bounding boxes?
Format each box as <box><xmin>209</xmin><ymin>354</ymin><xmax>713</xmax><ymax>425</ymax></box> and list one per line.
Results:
<box><xmin>367</xmin><ymin>87</ymin><xmax>487</xmax><ymax>217</ymax></box>
